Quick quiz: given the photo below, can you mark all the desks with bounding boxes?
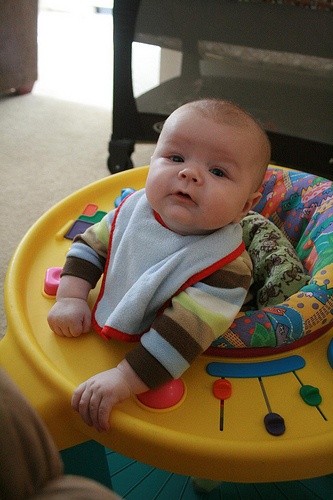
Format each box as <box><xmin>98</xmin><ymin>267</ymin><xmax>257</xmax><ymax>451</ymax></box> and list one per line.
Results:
<box><xmin>107</xmin><ymin>0</ymin><xmax>333</xmax><ymax>173</ymax></box>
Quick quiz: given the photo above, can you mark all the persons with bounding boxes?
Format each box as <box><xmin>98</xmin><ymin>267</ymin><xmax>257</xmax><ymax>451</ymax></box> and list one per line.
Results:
<box><xmin>46</xmin><ymin>98</ymin><xmax>272</xmax><ymax>433</ymax></box>
<box><xmin>0</xmin><ymin>367</ymin><xmax>124</xmax><ymax>500</ymax></box>
